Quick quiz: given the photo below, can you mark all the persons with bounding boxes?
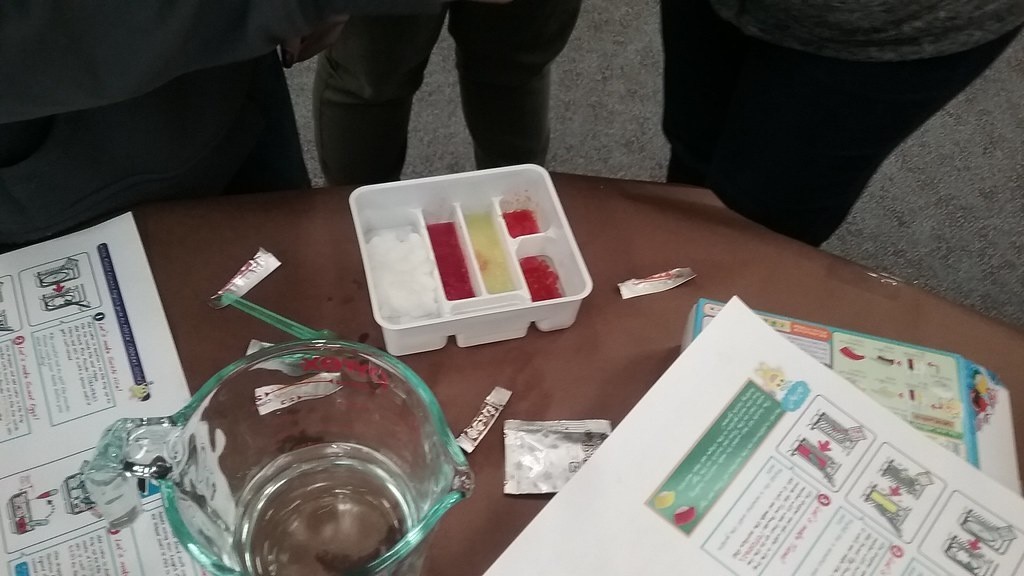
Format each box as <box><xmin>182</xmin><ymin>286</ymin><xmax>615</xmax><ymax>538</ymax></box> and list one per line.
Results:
<box><xmin>0</xmin><ymin>0</ymin><xmax>353</xmax><ymax>257</ymax></box>
<box><xmin>660</xmin><ymin>1</ymin><xmax>1024</xmax><ymax>248</ymax></box>
<box><xmin>312</xmin><ymin>0</ymin><xmax>583</xmax><ymax>187</ymax></box>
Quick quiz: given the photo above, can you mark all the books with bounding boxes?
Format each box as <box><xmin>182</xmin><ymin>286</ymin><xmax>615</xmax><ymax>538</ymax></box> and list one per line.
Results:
<box><xmin>680</xmin><ymin>297</ymin><xmax>1023</xmax><ymax>495</ymax></box>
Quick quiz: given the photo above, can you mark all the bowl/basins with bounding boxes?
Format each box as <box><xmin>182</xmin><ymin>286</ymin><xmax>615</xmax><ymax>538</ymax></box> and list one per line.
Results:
<box><xmin>348</xmin><ymin>163</ymin><xmax>595</xmax><ymax>357</ymax></box>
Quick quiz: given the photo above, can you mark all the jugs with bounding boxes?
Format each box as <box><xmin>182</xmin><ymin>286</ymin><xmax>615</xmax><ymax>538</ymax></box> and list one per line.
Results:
<box><xmin>83</xmin><ymin>337</ymin><xmax>474</xmax><ymax>576</ymax></box>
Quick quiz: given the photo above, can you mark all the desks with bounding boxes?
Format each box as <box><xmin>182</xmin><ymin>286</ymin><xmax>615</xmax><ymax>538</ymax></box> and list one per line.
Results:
<box><xmin>117</xmin><ymin>172</ymin><xmax>1024</xmax><ymax>576</ymax></box>
